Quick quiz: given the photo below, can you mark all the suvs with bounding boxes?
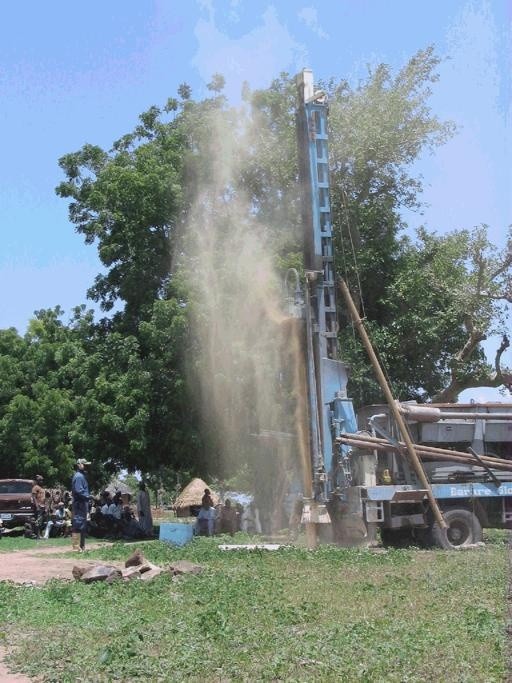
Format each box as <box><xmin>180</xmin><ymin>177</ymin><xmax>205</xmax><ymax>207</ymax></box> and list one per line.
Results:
<box><xmin>0</xmin><ymin>478</ymin><xmax>39</xmax><ymax>530</ymax></box>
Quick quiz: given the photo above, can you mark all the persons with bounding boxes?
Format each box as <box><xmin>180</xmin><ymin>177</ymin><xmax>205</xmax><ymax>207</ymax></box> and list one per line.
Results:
<box><xmin>70</xmin><ymin>458</ymin><xmax>93</xmax><ymax>552</ymax></box>
<box><xmin>31</xmin><ymin>475</ymin><xmax>154</xmax><ymax>540</ymax></box>
<box><xmin>194</xmin><ymin>489</ymin><xmax>262</xmax><ymax>536</ymax></box>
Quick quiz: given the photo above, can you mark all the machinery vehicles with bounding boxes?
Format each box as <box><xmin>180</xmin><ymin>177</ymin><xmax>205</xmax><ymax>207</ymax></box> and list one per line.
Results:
<box><xmin>293</xmin><ymin>69</ymin><xmax>512</xmax><ymax>548</ymax></box>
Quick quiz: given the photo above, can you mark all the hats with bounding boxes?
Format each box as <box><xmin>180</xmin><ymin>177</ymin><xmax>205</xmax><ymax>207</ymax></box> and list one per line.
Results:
<box><xmin>78</xmin><ymin>458</ymin><xmax>91</xmax><ymax>465</ymax></box>
